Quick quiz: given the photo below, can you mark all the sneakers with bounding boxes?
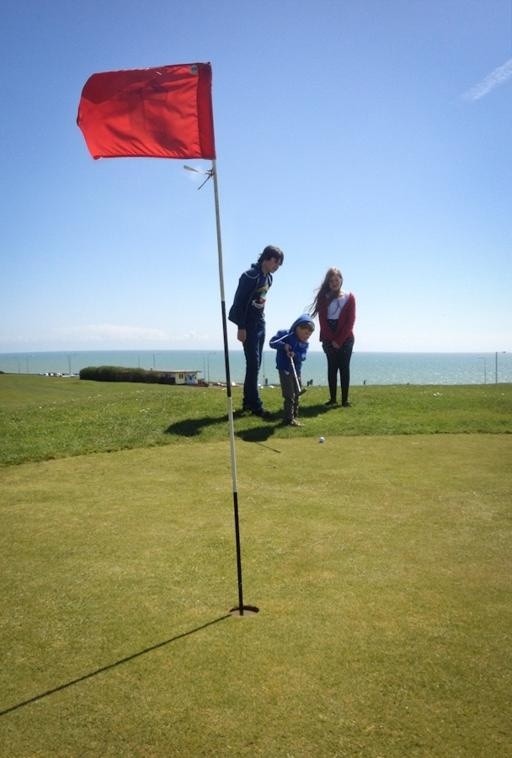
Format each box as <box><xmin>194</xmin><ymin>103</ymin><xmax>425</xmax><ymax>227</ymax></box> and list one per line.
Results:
<box><xmin>325</xmin><ymin>397</ymin><xmax>350</xmax><ymax>406</ymax></box>
<box><xmin>242</xmin><ymin>402</ymin><xmax>269</xmax><ymax>415</ymax></box>
<box><xmin>284</xmin><ymin>414</ymin><xmax>303</xmax><ymax>426</ymax></box>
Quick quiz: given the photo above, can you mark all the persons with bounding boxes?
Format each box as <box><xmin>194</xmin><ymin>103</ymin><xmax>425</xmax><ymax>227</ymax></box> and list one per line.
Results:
<box><xmin>227</xmin><ymin>242</ymin><xmax>284</xmax><ymax>415</ymax></box>
<box><xmin>303</xmin><ymin>266</ymin><xmax>358</xmax><ymax>409</ymax></box>
<box><xmin>268</xmin><ymin>313</ymin><xmax>316</xmax><ymax>428</ymax></box>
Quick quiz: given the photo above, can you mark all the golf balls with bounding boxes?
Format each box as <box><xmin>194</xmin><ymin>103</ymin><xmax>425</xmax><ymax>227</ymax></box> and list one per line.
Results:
<box><xmin>319</xmin><ymin>437</ymin><xmax>325</xmax><ymax>442</ymax></box>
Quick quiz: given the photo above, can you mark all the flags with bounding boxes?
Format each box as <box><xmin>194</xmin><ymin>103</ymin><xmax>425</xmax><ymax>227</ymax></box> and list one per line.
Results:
<box><xmin>75</xmin><ymin>55</ymin><xmax>216</xmax><ymax>162</ymax></box>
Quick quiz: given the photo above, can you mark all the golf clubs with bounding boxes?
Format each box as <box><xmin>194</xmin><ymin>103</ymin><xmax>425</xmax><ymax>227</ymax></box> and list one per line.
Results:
<box><xmin>289</xmin><ymin>346</ymin><xmax>308</xmax><ymax>395</ymax></box>
